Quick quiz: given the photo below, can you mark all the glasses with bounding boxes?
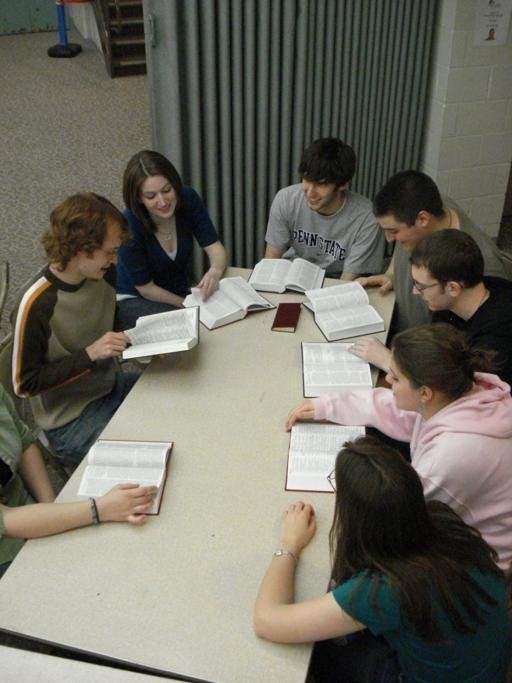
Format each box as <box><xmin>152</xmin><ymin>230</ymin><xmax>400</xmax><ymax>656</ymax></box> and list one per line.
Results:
<box><xmin>413</xmin><ymin>281</ymin><xmax>439</xmax><ymax>291</ymax></box>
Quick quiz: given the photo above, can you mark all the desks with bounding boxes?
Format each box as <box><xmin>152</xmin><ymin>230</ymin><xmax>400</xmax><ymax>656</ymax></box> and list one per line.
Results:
<box><xmin>0</xmin><ymin>263</ymin><xmax>399</xmax><ymax>683</ymax></box>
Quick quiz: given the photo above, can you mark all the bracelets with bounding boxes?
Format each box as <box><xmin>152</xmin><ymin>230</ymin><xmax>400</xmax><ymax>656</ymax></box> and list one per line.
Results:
<box><xmin>88</xmin><ymin>499</ymin><xmax>101</xmax><ymax>526</ymax></box>
<box><xmin>272</xmin><ymin>548</ymin><xmax>297</xmax><ymax>564</ymax></box>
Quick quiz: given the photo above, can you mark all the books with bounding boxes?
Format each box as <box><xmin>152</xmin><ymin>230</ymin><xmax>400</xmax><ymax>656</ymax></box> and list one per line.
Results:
<box><xmin>248</xmin><ymin>255</ymin><xmax>326</xmax><ymax>294</ymax></box>
<box><xmin>123</xmin><ymin>305</ymin><xmax>200</xmax><ymax>360</ymax></box>
<box><xmin>77</xmin><ymin>440</ymin><xmax>175</xmax><ymax>515</ymax></box>
<box><xmin>269</xmin><ymin>301</ymin><xmax>301</xmax><ymax>332</ymax></box>
<box><xmin>285</xmin><ymin>421</ymin><xmax>366</xmax><ymax>493</ymax></box>
<box><xmin>300</xmin><ymin>281</ymin><xmax>386</xmax><ymax>340</ymax></box>
<box><xmin>300</xmin><ymin>340</ymin><xmax>376</xmax><ymax>398</ymax></box>
<box><xmin>182</xmin><ymin>275</ymin><xmax>276</xmax><ymax>330</ymax></box>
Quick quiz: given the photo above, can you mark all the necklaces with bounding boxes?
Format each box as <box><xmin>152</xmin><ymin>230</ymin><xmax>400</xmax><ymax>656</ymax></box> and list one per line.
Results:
<box><xmin>158</xmin><ymin>226</ymin><xmax>177</xmax><ymax>242</ymax></box>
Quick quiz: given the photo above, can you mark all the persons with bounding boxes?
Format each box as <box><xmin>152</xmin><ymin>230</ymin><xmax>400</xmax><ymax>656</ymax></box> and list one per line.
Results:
<box><xmin>282</xmin><ymin>322</ymin><xmax>511</xmax><ymax>579</ymax></box>
<box><xmin>113</xmin><ymin>148</ymin><xmax>229</xmax><ymax>330</ymax></box>
<box><xmin>0</xmin><ymin>379</ymin><xmax>160</xmax><ymax>579</ymax></box>
<box><xmin>11</xmin><ymin>192</ymin><xmax>130</xmax><ymax>471</ymax></box>
<box><xmin>254</xmin><ymin>430</ymin><xmax>511</xmax><ymax>683</ymax></box>
<box><xmin>262</xmin><ymin>135</ymin><xmax>386</xmax><ymax>282</ymax></box>
<box><xmin>345</xmin><ymin>227</ymin><xmax>509</xmax><ymax>389</ymax></box>
<box><xmin>354</xmin><ymin>169</ymin><xmax>511</xmax><ymax>328</ymax></box>
